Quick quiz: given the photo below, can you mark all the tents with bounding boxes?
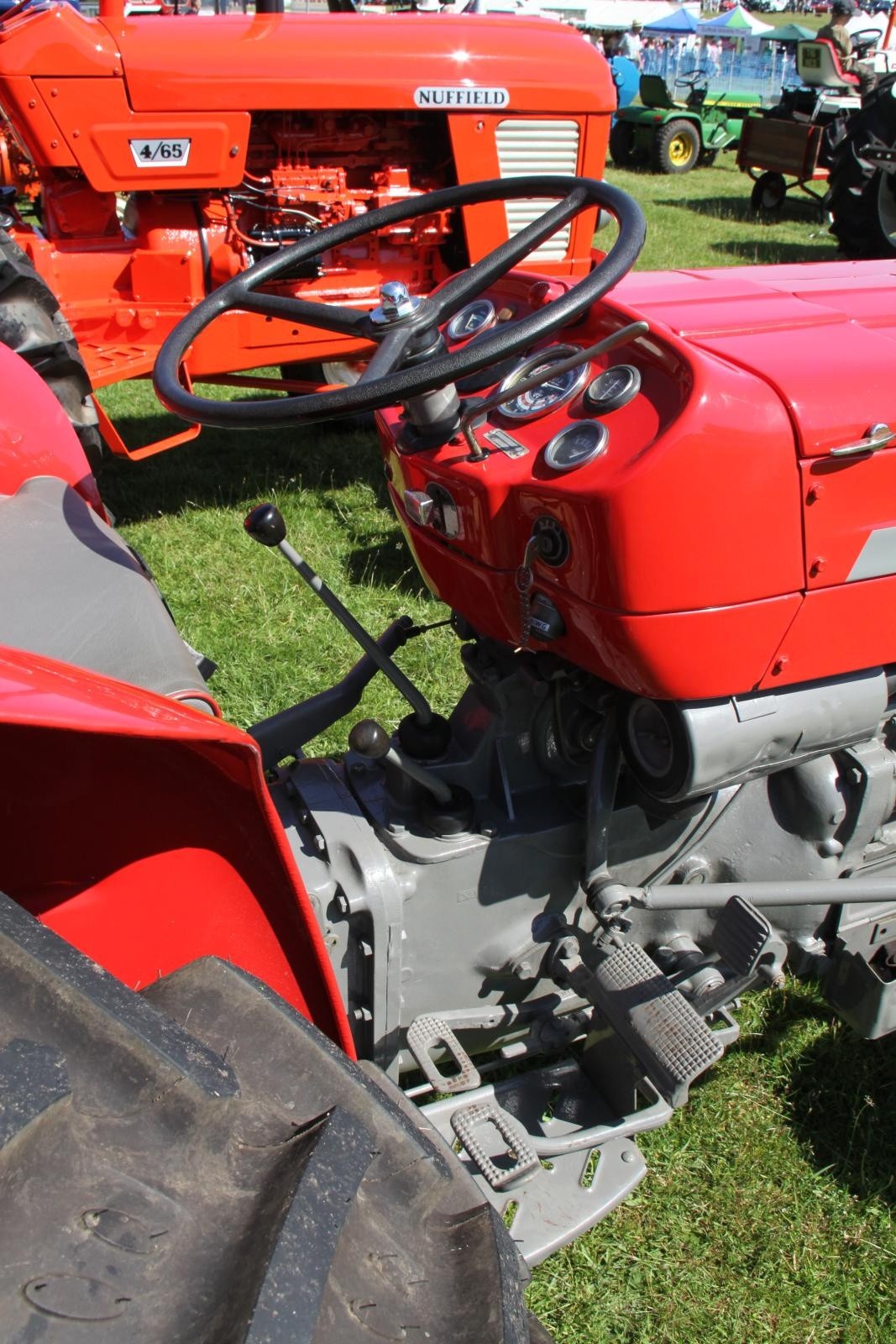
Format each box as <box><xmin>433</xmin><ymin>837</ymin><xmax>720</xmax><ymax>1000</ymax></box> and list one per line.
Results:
<box><xmin>640</xmin><ymin>8</ymin><xmax>705</xmax><ymax>101</ymax></box>
<box><xmin>758</xmin><ymin>23</ymin><xmax>817</xmax><ymax>94</ymax></box>
<box><xmin>691</xmin><ymin>4</ymin><xmax>775</xmax><ymax>92</ymax></box>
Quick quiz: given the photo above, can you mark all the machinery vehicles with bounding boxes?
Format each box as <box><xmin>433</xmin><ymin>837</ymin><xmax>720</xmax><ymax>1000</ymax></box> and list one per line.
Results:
<box><xmin>0</xmin><ymin>4</ymin><xmax>895</xmax><ymax>1343</ymax></box>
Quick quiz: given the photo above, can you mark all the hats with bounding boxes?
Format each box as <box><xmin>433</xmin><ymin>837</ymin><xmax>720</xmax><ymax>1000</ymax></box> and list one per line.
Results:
<box><xmin>830</xmin><ymin>0</ymin><xmax>854</xmax><ymax>13</ymax></box>
<box><xmin>632</xmin><ymin>20</ymin><xmax>644</xmax><ymax>27</ymax></box>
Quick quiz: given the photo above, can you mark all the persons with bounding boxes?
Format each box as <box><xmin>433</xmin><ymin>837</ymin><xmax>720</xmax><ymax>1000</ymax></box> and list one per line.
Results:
<box><xmin>639</xmin><ymin>35</ymin><xmax>797</xmax><ymax>85</ymax></box>
<box><xmin>818</xmin><ymin>0</ymin><xmax>878</xmax><ymax>102</ymax></box>
<box><xmin>594</xmin><ymin>36</ymin><xmax>605</xmax><ymax>56</ymax></box>
<box><xmin>615</xmin><ymin>19</ymin><xmax>644</xmax><ymax>72</ymax></box>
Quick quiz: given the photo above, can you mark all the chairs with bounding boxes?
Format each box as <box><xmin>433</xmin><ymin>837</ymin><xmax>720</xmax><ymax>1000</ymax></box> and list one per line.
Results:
<box><xmin>638</xmin><ymin>74</ymin><xmax>689</xmax><ymax>110</ymax></box>
<box><xmin>796</xmin><ymin>37</ymin><xmax>861</xmax><ymax>89</ymax></box>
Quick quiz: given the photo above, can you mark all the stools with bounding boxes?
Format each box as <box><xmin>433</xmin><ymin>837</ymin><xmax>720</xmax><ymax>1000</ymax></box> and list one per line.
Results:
<box><xmin>0</xmin><ymin>475</ymin><xmax>219</xmax><ymax>721</ymax></box>
